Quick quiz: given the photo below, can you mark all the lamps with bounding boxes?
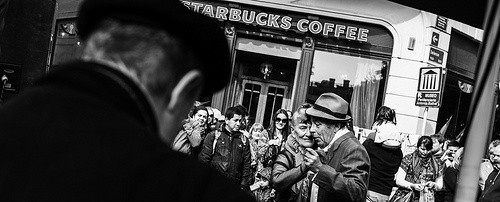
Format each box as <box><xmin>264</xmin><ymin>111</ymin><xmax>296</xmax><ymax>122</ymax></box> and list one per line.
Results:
<box><xmin>260</xmin><ymin>62</ymin><xmax>273</xmax><ymax>79</ymax></box>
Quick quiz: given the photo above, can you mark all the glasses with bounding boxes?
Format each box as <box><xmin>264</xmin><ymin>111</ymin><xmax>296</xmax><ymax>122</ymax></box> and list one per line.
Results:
<box><xmin>274</xmin><ymin>118</ymin><xmax>287</xmax><ymax>124</ymax></box>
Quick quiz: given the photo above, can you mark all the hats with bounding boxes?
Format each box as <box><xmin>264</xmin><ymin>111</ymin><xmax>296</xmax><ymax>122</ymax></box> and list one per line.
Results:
<box><xmin>76</xmin><ymin>0</ymin><xmax>232</xmax><ymax>99</ymax></box>
<box><xmin>306</xmin><ymin>93</ymin><xmax>351</xmax><ymax>120</ymax></box>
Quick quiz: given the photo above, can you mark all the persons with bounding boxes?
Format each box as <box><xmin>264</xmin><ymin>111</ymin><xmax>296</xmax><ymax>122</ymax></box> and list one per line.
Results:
<box><xmin>0</xmin><ymin>0</ymin><xmax>260</xmax><ymax>202</ymax></box>
<box><xmin>270</xmin><ymin>105</ymin><xmax>327</xmax><ymax>202</ymax></box>
<box><xmin>172</xmin><ymin>101</ymin><xmax>293</xmax><ymax>202</ymax></box>
<box><xmin>388</xmin><ymin>136</ymin><xmax>443</xmax><ymax>202</ymax></box>
<box><xmin>427</xmin><ymin>133</ymin><xmax>500</xmax><ymax>202</ymax></box>
<box><xmin>288</xmin><ymin>93</ymin><xmax>371</xmax><ymax>202</ymax></box>
<box><xmin>362</xmin><ymin>106</ymin><xmax>403</xmax><ymax>202</ymax></box>
